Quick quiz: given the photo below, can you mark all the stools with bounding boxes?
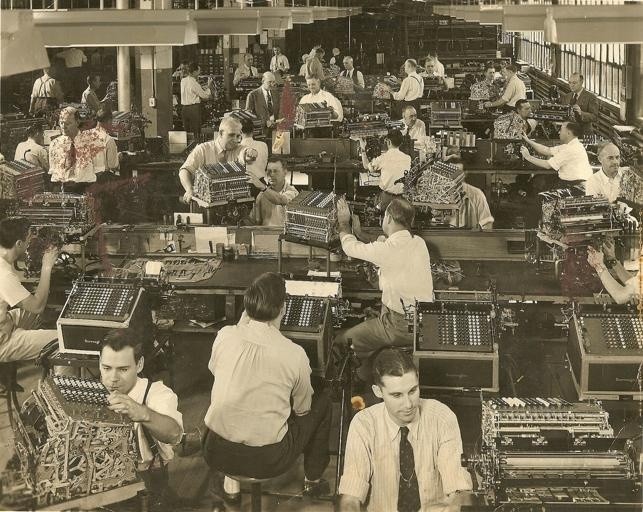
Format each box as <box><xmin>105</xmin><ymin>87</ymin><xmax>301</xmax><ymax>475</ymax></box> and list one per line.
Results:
<box><xmin>389</xmin><ymin>345</ymin><xmax>414</xmax><ymax>354</ymax></box>
<box><xmin>218</xmin><ymin>459</ymin><xmax>297</xmax><ymax>512</ymax></box>
<box><xmin>0</xmin><ymin>361</ymin><xmax>20</xmax><ymax>427</ymax></box>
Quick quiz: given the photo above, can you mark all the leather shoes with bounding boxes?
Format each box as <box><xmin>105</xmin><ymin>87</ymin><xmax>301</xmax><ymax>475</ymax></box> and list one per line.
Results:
<box><xmin>213</xmin><ymin>472</ymin><xmax>241</xmax><ymax>506</ymax></box>
<box><xmin>304</xmin><ymin>479</ymin><xmax>331</xmax><ymax>496</ymax></box>
<box><xmin>1</xmin><ymin>370</ymin><xmax>24</xmax><ymax>392</ymax></box>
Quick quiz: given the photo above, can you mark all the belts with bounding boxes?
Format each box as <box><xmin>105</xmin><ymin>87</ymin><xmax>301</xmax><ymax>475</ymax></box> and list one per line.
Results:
<box><xmin>384</xmin><ymin>191</ymin><xmax>403</xmax><ymax>197</ymax></box>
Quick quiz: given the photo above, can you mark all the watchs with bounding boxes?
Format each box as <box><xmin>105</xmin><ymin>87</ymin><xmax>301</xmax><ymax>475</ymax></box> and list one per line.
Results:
<box><xmin>261</xmin><ymin>184</ymin><xmax>268</xmax><ymax>192</ymax></box>
<box><xmin>596</xmin><ymin>267</ymin><xmax>608</xmax><ymax>276</ymax></box>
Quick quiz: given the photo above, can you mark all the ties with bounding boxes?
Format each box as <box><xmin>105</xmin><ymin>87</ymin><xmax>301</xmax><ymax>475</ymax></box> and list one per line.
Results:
<box><xmin>347</xmin><ymin>71</ymin><xmax>352</xmax><ymax>78</ymax></box>
<box><xmin>248</xmin><ymin>68</ymin><xmax>254</xmax><ymax>77</ymax></box>
<box><xmin>405</xmin><ymin>128</ymin><xmax>411</xmax><ymax>141</ymax></box>
<box><xmin>275</xmin><ymin>55</ymin><xmax>279</xmax><ymax>70</ymax></box>
<box><xmin>219</xmin><ymin>150</ymin><xmax>227</xmax><ymax>163</ymax></box>
<box><xmin>266</xmin><ymin>90</ymin><xmax>273</xmax><ymax>116</ymax></box>
<box><xmin>398</xmin><ymin>428</ymin><xmax>421</xmax><ymax>512</ymax></box>
<box><xmin>67</xmin><ymin>144</ymin><xmax>79</xmax><ymax>178</ymax></box>
<box><xmin>526</xmin><ymin>120</ymin><xmax>532</xmax><ymax>135</ymax></box>
<box><xmin>571</xmin><ymin>93</ymin><xmax>579</xmax><ymax>103</ymax></box>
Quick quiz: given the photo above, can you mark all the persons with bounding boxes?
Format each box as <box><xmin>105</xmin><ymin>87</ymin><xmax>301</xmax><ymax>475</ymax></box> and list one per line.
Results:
<box><xmin>245</xmin><ymin>155</ymin><xmax>297</xmax><ymax>224</ymax></box>
<box><xmin>393</xmin><ymin>105</ymin><xmax>425</xmax><ymax>164</ymax></box>
<box><xmin>180</xmin><ymin>64</ymin><xmax>213</xmax><ymax>136</ymax></box>
<box><xmin>478</xmin><ymin>54</ymin><xmax>527</xmax><ymax>82</ymax></box>
<box><xmin>339</xmin><ymin>56</ymin><xmax>365</xmax><ymax>92</ymax></box>
<box><xmin>337</xmin><ymin>347</ymin><xmax>472</xmax><ymax>508</ymax></box>
<box><xmin>238</xmin><ymin>119</ymin><xmax>267</xmax><ymax>196</ymax></box>
<box><xmin>269</xmin><ymin>46</ymin><xmax>289</xmax><ymax>75</ymax></box>
<box><xmin>331</xmin><ymin>199</ymin><xmax>435</xmax><ymax>395</ymax></box>
<box><xmin>247</xmin><ymin>72</ymin><xmax>279</xmax><ymax>135</ymax></box>
<box><xmin>56</xmin><ymin>48</ymin><xmax>86</xmax><ymax>99</ymax></box>
<box><xmin>513</xmin><ymin>100</ymin><xmax>536</xmax><ymax>137</ymax></box>
<box><xmin>29</xmin><ymin>66</ymin><xmax>62</xmax><ymax>119</ymax></box>
<box><xmin>553</xmin><ymin>72</ymin><xmax>597</xmax><ymax>137</ymax></box>
<box><xmin>587</xmin><ymin>241</ymin><xmax>641</xmax><ymax>305</ymax></box>
<box><xmin>384</xmin><ymin>58</ymin><xmax>422</xmax><ymax>120</ymax></box>
<box><xmin>48</xmin><ymin>107</ymin><xmax>99</xmax><ymax>195</ymax></box>
<box><xmin>587</xmin><ymin>142</ymin><xmax>633</xmax><ymax>208</ymax></box>
<box><xmin>202</xmin><ymin>274</ymin><xmax>332</xmax><ymax>507</ymax></box>
<box><xmin>298</xmin><ymin>45</ymin><xmax>334</xmax><ymax>87</ymax></box>
<box><xmin>80</xmin><ymin>72</ymin><xmax>109</xmax><ymax>115</ymax></box>
<box><xmin>1</xmin><ymin>219</ymin><xmax>59</xmax><ymax>387</ymax></box>
<box><xmin>519</xmin><ymin>122</ymin><xmax>592</xmax><ymax>226</ymax></box>
<box><xmin>77</xmin><ymin>111</ymin><xmax>119</xmax><ymax>182</ymax></box>
<box><xmin>98</xmin><ymin>332</ymin><xmax>184</xmax><ymax>507</ymax></box>
<box><xmin>429</xmin><ymin>51</ymin><xmax>445</xmax><ymax>78</ymax></box>
<box><xmin>180</xmin><ymin>117</ymin><xmax>257</xmax><ymax>209</ymax></box>
<box><xmin>299</xmin><ymin>75</ymin><xmax>343</xmax><ymax>136</ymax></box>
<box><xmin>478</xmin><ymin>65</ymin><xmax>525</xmax><ymax>118</ymax></box>
<box><xmin>234</xmin><ymin>52</ymin><xmax>257</xmax><ymax>88</ymax></box>
<box><xmin>15</xmin><ymin>124</ymin><xmax>47</xmax><ymax>171</ymax></box>
<box><xmin>442</xmin><ymin>157</ymin><xmax>494</xmax><ymax>231</ymax></box>
<box><xmin>360</xmin><ymin>129</ymin><xmax>410</xmax><ymax>215</ymax></box>
<box><xmin>415</xmin><ymin>60</ymin><xmax>439</xmax><ymax>80</ymax></box>
